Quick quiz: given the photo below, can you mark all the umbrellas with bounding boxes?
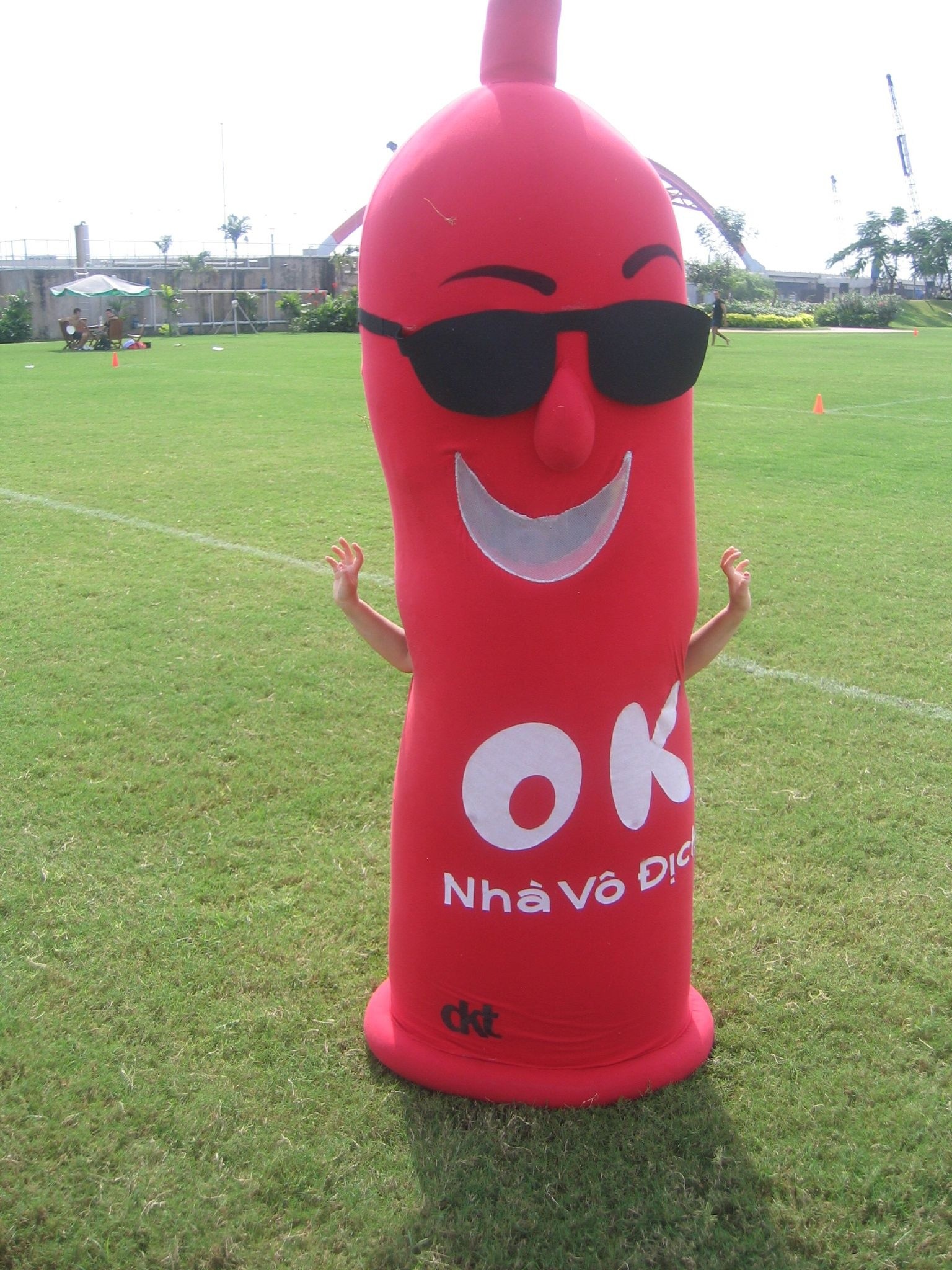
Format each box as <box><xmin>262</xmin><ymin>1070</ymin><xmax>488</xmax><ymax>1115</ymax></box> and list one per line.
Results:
<box><xmin>50</xmin><ymin>274</ymin><xmax>151</xmax><ymax>316</ymax></box>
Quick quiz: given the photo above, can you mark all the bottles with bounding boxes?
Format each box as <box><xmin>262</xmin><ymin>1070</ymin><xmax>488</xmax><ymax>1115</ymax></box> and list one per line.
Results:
<box><xmin>99</xmin><ymin>316</ymin><xmax>102</xmax><ymax>326</ymax></box>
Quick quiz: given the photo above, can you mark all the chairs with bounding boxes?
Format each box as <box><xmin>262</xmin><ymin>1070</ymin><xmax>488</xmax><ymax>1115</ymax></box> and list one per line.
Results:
<box><xmin>59</xmin><ymin>317</ymin><xmax>122</xmax><ymax>351</ymax></box>
<box><xmin>127</xmin><ymin>317</ymin><xmax>147</xmax><ymax>342</ymax></box>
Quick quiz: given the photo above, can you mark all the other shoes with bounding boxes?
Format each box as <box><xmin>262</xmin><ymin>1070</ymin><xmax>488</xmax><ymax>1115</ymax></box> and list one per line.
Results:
<box><xmin>726</xmin><ymin>338</ymin><xmax>730</xmax><ymax>347</ymax></box>
<box><xmin>73</xmin><ymin>347</ymin><xmax>81</xmax><ymax>350</ymax></box>
<box><xmin>83</xmin><ymin>346</ymin><xmax>90</xmax><ymax>351</ymax></box>
<box><xmin>90</xmin><ymin>347</ymin><xmax>94</xmax><ymax>350</ymax></box>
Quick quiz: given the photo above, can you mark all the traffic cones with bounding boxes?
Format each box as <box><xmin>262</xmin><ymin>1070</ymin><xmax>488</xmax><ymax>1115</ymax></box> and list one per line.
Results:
<box><xmin>914</xmin><ymin>328</ymin><xmax>917</xmax><ymax>336</ymax></box>
<box><xmin>813</xmin><ymin>394</ymin><xmax>824</xmax><ymax>413</ymax></box>
<box><xmin>113</xmin><ymin>352</ymin><xmax>119</xmax><ymax>367</ymax></box>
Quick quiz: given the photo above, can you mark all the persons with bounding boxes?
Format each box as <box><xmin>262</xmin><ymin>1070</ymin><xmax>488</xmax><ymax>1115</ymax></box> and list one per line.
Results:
<box><xmin>709</xmin><ymin>290</ymin><xmax>731</xmax><ymax>346</ymax></box>
<box><xmin>325</xmin><ymin>0</ymin><xmax>750</xmax><ymax>1105</ymax></box>
<box><xmin>58</xmin><ymin>308</ymin><xmax>96</xmax><ymax>351</ymax></box>
<box><xmin>94</xmin><ymin>309</ymin><xmax>117</xmax><ymax>350</ymax></box>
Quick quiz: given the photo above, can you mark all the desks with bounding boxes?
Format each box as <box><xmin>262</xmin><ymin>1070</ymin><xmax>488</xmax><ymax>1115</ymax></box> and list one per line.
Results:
<box><xmin>85</xmin><ymin>325</ymin><xmax>103</xmax><ymax>346</ymax></box>
